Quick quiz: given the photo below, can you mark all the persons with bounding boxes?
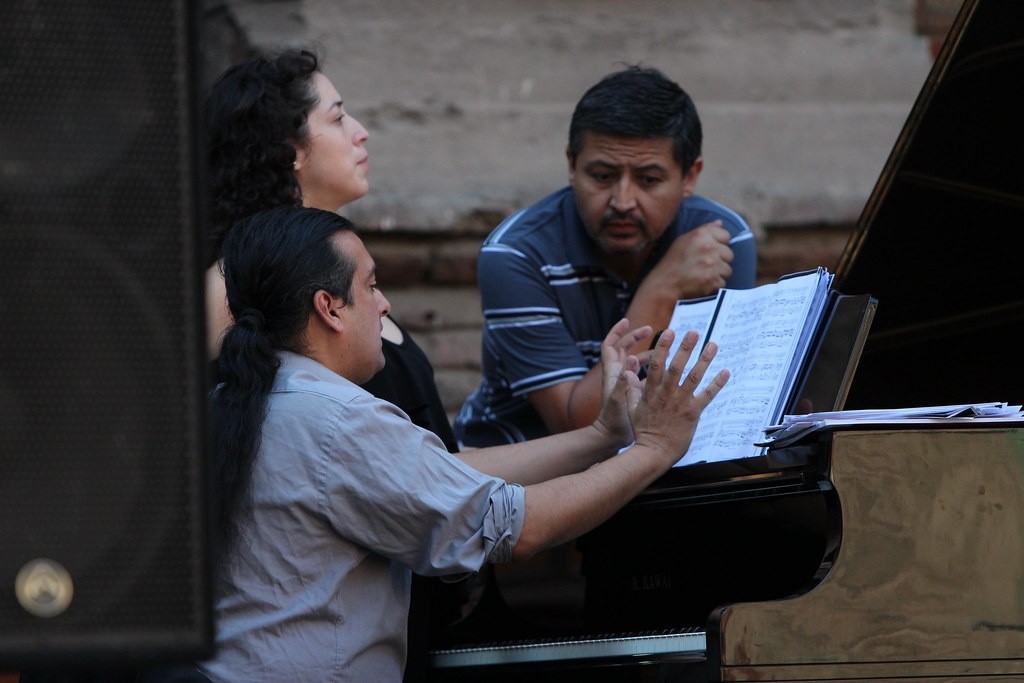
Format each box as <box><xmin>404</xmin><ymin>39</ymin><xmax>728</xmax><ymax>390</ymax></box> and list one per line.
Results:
<box><xmin>204</xmin><ymin>45</ymin><xmax>478</xmax><ymax>451</ymax></box>
<box><xmin>181</xmin><ymin>214</ymin><xmax>729</xmax><ymax>683</ymax></box>
<box><xmin>457</xmin><ymin>64</ymin><xmax>758</xmax><ymax>451</ymax></box>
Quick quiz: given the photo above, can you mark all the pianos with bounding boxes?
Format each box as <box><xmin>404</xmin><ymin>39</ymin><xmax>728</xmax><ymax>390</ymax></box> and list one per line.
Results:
<box><xmin>397</xmin><ymin>0</ymin><xmax>1024</xmax><ymax>683</ymax></box>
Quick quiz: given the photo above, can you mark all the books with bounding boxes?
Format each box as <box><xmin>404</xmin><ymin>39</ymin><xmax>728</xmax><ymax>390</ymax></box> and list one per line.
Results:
<box><xmin>752</xmin><ymin>402</ymin><xmax>1024</xmax><ymax>449</ymax></box>
<box><xmin>615</xmin><ymin>267</ymin><xmax>835</xmax><ymax>465</ymax></box>
